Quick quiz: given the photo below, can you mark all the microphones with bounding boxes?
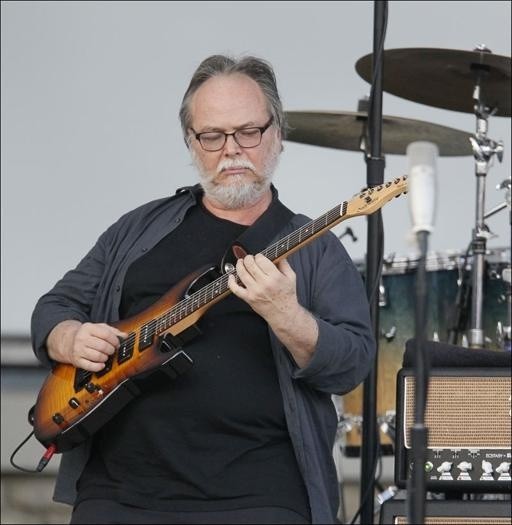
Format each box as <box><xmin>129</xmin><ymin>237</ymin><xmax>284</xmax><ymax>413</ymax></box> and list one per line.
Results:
<box><xmin>407</xmin><ymin>141</ymin><xmax>438</xmax><ymax>255</ymax></box>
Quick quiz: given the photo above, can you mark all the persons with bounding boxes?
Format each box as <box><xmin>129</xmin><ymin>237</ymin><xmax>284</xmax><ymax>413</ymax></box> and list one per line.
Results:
<box><xmin>29</xmin><ymin>54</ymin><xmax>379</xmax><ymax>525</ymax></box>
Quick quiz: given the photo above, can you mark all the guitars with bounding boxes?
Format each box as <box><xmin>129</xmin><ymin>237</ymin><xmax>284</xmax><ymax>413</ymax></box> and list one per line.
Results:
<box><xmin>33</xmin><ymin>174</ymin><xmax>409</xmax><ymax>452</ymax></box>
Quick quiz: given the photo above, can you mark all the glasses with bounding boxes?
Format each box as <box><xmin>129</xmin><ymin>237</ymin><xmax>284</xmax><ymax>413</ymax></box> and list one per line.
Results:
<box><xmin>191</xmin><ymin>117</ymin><xmax>275</xmax><ymax>151</ymax></box>
